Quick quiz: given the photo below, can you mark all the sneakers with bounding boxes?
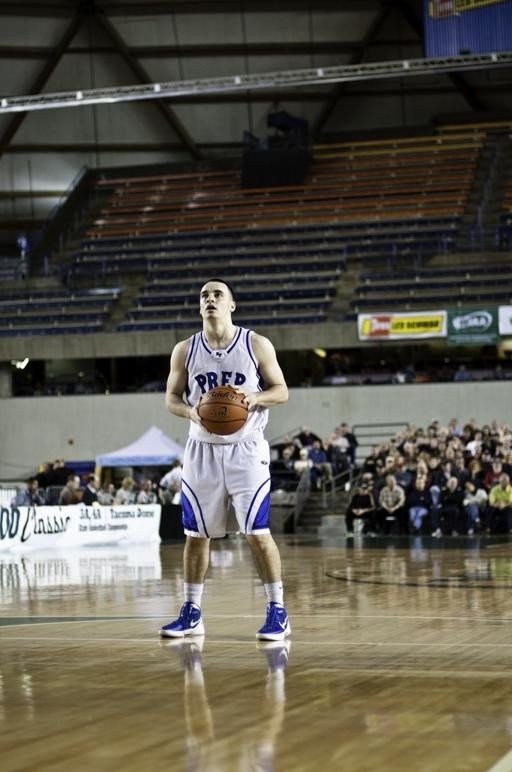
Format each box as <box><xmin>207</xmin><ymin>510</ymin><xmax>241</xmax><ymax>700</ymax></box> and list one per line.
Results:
<box><xmin>158</xmin><ymin>601</ymin><xmax>206</xmax><ymax>637</ymax></box>
<box><xmin>158</xmin><ymin>636</ymin><xmax>206</xmax><ymax>673</ymax></box>
<box><xmin>255</xmin><ymin>601</ymin><xmax>292</xmax><ymax>642</ymax></box>
<box><xmin>255</xmin><ymin>638</ymin><xmax>292</xmax><ymax>679</ymax></box>
<box><xmin>345</xmin><ymin>527</ymin><xmax>512</xmax><ymax>540</ymax></box>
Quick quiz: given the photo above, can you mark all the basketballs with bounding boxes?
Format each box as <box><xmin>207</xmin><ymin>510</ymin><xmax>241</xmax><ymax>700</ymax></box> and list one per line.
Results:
<box><xmin>198</xmin><ymin>387</ymin><xmax>249</xmax><ymax>435</ymax></box>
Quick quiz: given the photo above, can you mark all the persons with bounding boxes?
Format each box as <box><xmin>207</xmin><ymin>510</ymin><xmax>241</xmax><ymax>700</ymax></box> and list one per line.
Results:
<box><xmin>25</xmin><ymin>459</ymin><xmax>182</xmax><ymax>510</ymax></box>
<box><xmin>161</xmin><ymin>278</ymin><xmax>292</xmax><ymax>642</ymax></box>
<box><xmin>273</xmin><ymin>420</ymin><xmax>512</xmax><ymax>539</ymax></box>
<box><xmin>273</xmin><ymin>349</ymin><xmax>512</xmax><ymax>387</ymax></box>
<box><xmin>9</xmin><ymin>371</ymin><xmax>167</xmax><ymax>395</ymax></box>
<box><xmin>160</xmin><ymin>638</ymin><xmax>291</xmax><ymax>772</ymax></box>
